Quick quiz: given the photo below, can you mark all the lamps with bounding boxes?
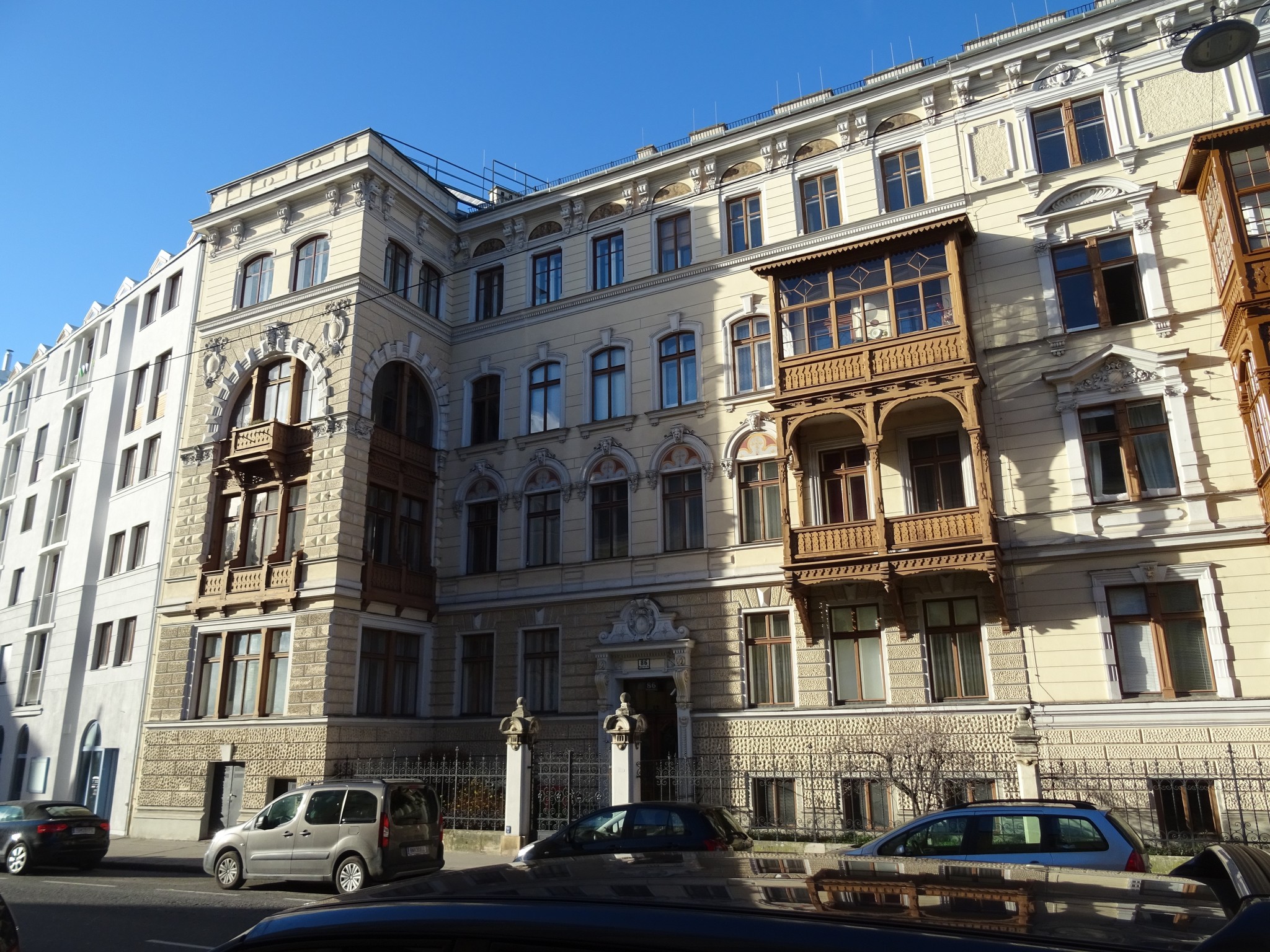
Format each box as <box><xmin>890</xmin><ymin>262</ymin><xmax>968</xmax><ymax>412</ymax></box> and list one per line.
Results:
<box><xmin>1182</xmin><ymin>6</ymin><xmax>1260</xmax><ymax>73</ymax></box>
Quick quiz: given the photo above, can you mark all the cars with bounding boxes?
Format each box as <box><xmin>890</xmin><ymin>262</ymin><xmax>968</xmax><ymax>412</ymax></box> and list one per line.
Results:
<box><xmin>512</xmin><ymin>800</ymin><xmax>754</xmax><ymax>864</ymax></box>
<box><xmin>0</xmin><ymin>800</ymin><xmax>112</xmax><ymax>876</ymax></box>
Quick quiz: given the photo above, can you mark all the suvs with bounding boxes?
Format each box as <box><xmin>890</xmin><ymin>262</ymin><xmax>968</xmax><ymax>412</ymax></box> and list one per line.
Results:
<box><xmin>203</xmin><ymin>777</ymin><xmax>445</xmax><ymax>895</ymax></box>
<box><xmin>212</xmin><ymin>840</ymin><xmax>1269</xmax><ymax>952</ymax></box>
<box><xmin>823</xmin><ymin>797</ymin><xmax>1155</xmax><ymax>877</ymax></box>
